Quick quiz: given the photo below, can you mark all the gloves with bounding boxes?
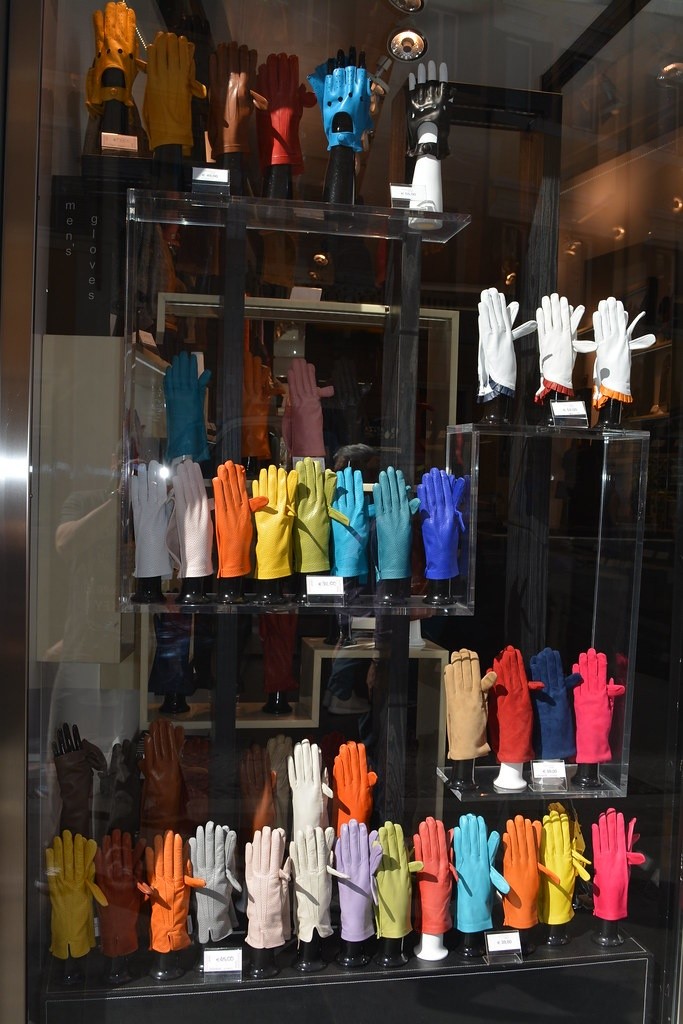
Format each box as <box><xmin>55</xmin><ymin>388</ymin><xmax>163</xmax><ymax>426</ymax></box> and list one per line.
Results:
<box><xmin>44</xmin><ymin>598</ymin><xmax>645</xmax><ymax>960</ymax></box>
<box><xmin>475</xmin><ymin>285</ymin><xmax>656</xmax><ymax>426</ymax></box>
<box><xmin>82</xmin><ymin>2</ymin><xmax>458</xmax><ymax>177</ymax></box>
<box><xmin>129</xmin><ymin>352</ymin><xmax>469</xmax><ymax>582</ymax></box>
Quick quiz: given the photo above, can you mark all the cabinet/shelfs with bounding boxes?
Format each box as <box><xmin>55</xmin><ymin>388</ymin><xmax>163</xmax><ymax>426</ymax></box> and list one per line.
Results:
<box><xmin>21</xmin><ymin>76</ymin><xmax>683</xmax><ymax>1024</ymax></box>
